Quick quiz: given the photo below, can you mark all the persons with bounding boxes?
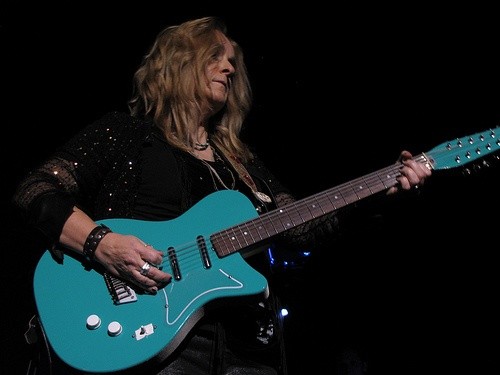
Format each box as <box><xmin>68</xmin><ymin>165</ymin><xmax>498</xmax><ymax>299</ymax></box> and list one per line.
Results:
<box><xmin>12</xmin><ymin>17</ymin><xmax>433</xmax><ymax>375</ymax></box>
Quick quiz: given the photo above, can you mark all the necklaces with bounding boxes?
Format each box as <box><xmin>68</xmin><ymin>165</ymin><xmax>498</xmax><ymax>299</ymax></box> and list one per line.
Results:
<box><xmin>183</xmin><ymin>136</ymin><xmax>211</xmax><ymax>151</ymax></box>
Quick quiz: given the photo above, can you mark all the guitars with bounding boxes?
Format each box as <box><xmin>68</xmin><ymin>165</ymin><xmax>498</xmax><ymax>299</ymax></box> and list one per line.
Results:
<box><xmin>32</xmin><ymin>126</ymin><xmax>500</xmax><ymax>373</ymax></box>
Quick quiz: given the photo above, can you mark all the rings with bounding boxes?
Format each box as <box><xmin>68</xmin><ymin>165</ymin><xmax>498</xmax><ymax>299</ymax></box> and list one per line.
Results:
<box><xmin>139</xmin><ymin>260</ymin><xmax>151</xmax><ymax>277</ymax></box>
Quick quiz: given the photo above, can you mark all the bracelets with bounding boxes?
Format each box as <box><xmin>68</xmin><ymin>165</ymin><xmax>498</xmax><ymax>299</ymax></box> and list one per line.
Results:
<box><xmin>81</xmin><ymin>222</ymin><xmax>112</xmax><ymax>272</ymax></box>
